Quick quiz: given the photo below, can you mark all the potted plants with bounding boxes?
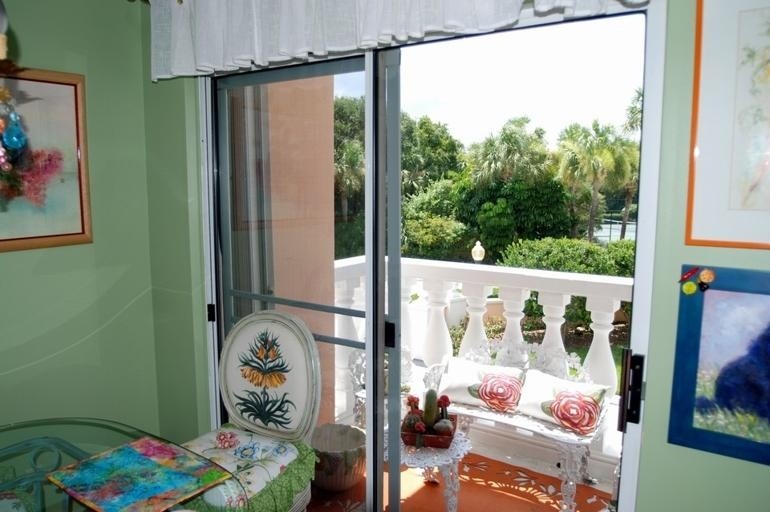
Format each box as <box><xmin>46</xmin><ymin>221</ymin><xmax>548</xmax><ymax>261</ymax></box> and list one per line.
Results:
<box><xmin>401</xmin><ymin>387</ymin><xmax>458</xmax><ymax>450</ymax></box>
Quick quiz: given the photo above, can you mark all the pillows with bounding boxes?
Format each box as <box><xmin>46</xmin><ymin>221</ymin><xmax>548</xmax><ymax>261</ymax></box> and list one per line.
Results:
<box><xmin>518</xmin><ymin>370</ymin><xmax>609</xmax><ymax>430</ymax></box>
<box><xmin>439</xmin><ymin>358</ymin><xmax>524</xmax><ymax>413</ymax></box>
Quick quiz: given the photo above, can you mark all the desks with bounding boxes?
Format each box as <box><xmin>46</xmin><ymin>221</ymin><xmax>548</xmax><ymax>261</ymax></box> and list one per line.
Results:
<box><xmin>424</xmin><ymin>340</ymin><xmax>604</xmax><ymax>511</ymax></box>
<box><xmin>1</xmin><ymin>415</ymin><xmax>249</xmax><ymax>512</ymax></box>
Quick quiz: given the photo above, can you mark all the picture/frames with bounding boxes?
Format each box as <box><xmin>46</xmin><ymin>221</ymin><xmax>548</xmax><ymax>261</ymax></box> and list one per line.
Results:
<box><xmin>0</xmin><ymin>64</ymin><xmax>95</xmax><ymax>251</ymax></box>
<box><xmin>666</xmin><ymin>264</ymin><xmax>770</xmax><ymax>467</ymax></box>
<box><xmin>685</xmin><ymin>0</ymin><xmax>770</xmax><ymax>249</ymax></box>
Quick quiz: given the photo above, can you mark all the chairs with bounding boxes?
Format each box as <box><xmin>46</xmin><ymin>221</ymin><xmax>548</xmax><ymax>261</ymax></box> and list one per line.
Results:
<box><xmin>177</xmin><ymin>310</ymin><xmax>322</xmax><ymax>512</ymax></box>
<box><xmin>346</xmin><ymin>335</ymin><xmax>470</xmax><ymax>512</ymax></box>
